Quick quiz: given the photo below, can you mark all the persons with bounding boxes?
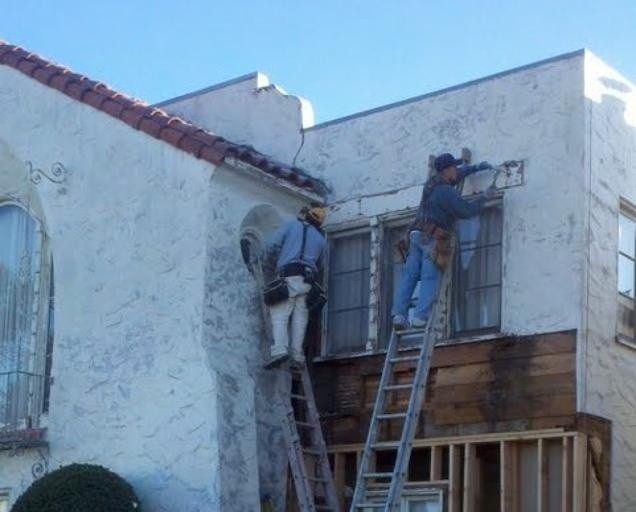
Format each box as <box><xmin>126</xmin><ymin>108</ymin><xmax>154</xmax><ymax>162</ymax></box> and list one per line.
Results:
<box><xmin>387</xmin><ymin>153</ymin><xmax>505</xmax><ymax>330</ymax></box>
<box><xmin>262</xmin><ymin>206</ymin><xmax>331</xmax><ymax>371</ymax></box>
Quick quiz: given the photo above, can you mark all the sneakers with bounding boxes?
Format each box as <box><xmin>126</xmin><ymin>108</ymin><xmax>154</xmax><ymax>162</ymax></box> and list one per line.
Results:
<box><xmin>264</xmin><ymin>353</ymin><xmax>288</xmax><ymax>370</ymax></box>
<box><xmin>393</xmin><ymin>314</ymin><xmax>426</xmax><ymax>330</ymax></box>
<box><xmin>289</xmin><ymin>360</ymin><xmax>302</xmax><ymax>371</ymax></box>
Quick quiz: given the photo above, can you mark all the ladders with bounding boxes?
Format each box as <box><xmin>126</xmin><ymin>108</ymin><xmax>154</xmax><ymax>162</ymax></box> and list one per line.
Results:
<box><xmin>349</xmin><ymin>147</ymin><xmax>473</xmax><ymax>512</ymax></box>
<box><xmin>249</xmin><ymin>242</ymin><xmax>341</xmax><ymax>512</ymax></box>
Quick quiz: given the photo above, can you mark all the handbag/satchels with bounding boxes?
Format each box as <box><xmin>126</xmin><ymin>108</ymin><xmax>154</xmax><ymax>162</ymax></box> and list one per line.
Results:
<box><xmin>305</xmin><ymin>282</ymin><xmax>328</xmax><ymax>313</ymax></box>
<box><xmin>263</xmin><ymin>280</ymin><xmax>288</xmax><ymax>306</ymax></box>
<box><xmin>432</xmin><ymin>238</ymin><xmax>450</xmax><ymax>266</ymax></box>
<box><xmin>391</xmin><ymin>237</ymin><xmax>408</xmax><ymax>263</ymax></box>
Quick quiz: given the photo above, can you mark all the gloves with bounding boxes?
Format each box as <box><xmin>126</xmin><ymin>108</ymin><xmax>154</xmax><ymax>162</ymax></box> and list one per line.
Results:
<box><xmin>475</xmin><ymin>161</ymin><xmax>491</xmax><ymax>171</ymax></box>
<box><xmin>482</xmin><ymin>186</ymin><xmax>499</xmax><ymax>199</ymax></box>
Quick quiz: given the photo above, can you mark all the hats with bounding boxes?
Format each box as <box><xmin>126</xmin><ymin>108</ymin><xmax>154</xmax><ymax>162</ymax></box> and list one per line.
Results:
<box><xmin>435</xmin><ymin>154</ymin><xmax>463</xmax><ymax>170</ymax></box>
<box><xmin>308</xmin><ymin>208</ymin><xmax>325</xmax><ymax>223</ymax></box>
